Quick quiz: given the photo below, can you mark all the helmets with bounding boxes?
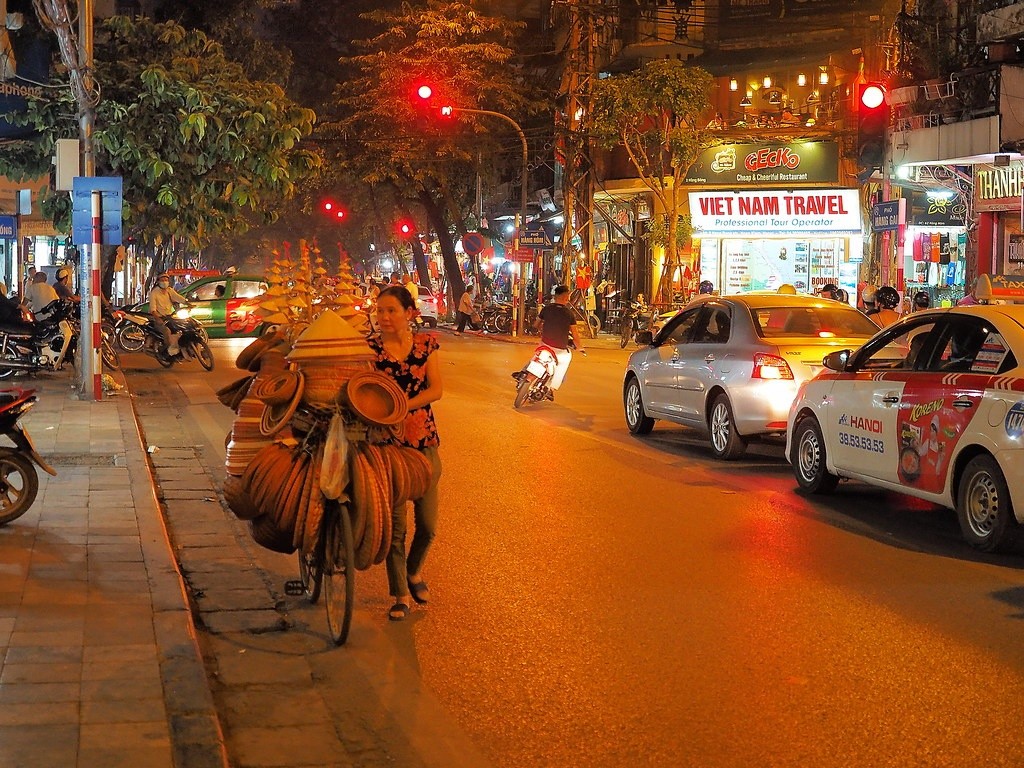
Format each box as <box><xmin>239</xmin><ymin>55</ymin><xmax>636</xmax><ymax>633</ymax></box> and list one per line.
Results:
<box><xmin>56</xmin><ymin>269</ymin><xmax>68</xmax><ymax>279</ymax></box>
<box><xmin>700</xmin><ymin>281</ymin><xmax>713</xmax><ymax>292</ymax></box>
<box><xmin>834</xmin><ymin>289</ymin><xmax>848</xmax><ymax>302</ymax></box>
<box><xmin>861</xmin><ymin>285</ymin><xmax>878</xmax><ymax>302</ymax></box>
<box><xmin>914</xmin><ymin>290</ymin><xmax>929</xmax><ymax>307</ymax></box>
<box><xmin>158</xmin><ymin>272</ymin><xmax>169</xmax><ymax>280</ymax></box>
<box><xmin>877</xmin><ymin>286</ymin><xmax>900</xmax><ymax>308</ymax></box>
<box><xmin>777</xmin><ymin>284</ymin><xmax>796</xmax><ymax>294</ymax></box>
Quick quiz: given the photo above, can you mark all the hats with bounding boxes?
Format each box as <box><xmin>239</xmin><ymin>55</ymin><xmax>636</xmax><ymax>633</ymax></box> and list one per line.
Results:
<box><xmin>822</xmin><ymin>284</ymin><xmax>838</xmax><ymax>292</ymax></box>
<box><xmin>284</xmin><ymin>310</ymin><xmax>376</xmax><ymax>404</ymax></box>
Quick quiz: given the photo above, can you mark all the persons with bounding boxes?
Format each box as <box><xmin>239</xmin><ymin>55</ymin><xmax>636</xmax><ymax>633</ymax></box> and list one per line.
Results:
<box><xmin>698</xmin><ymin>280</ymin><xmax>713</xmax><ymax>294</ymax></box>
<box><xmin>705</xmin><ymin>107</ymin><xmax>801</xmax><ymax>130</ymax></box>
<box><xmin>453</xmin><ymin>274</ymin><xmax>482</xmax><ymax>336</ymax></box>
<box><xmin>512</xmin><ymin>286</ymin><xmax>585</xmax><ymax>401</ymax></box>
<box><xmin>360</xmin><ymin>272</ymin><xmax>418</xmax><ymax>308</ymax></box>
<box><xmin>173</xmin><ymin>275</ymin><xmax>191</xmax><ymax>293</ymax></box>
<box><xmin>906</xmin><ymin>331</ymin><xmax>931</xmax><ymax>369</ymax></box>
<box><xmin>21</xmin><ymin>272</ymin><xmax>59</xmax><ymax>321</ymax></box>
<box><xmin>52</xmin><ymin>269</ymin><xmax>81</xmax><ymax>302</ymax></box>
<box><xmin>816</xmin><ymin>284</ymin><xmax>931</xmax><ymax>329</ymax></box>
<box><xmin>684</xmin><ymin>265</ymin><xmax>692</xmax><ymax>280</ymax></box>
<box><xmin>636</xmin><ymin>292</ymin><xmax>648</xmax><ymax>313</ymax></box>
<box><xmin>767</xmin><ymin>284</ymin><xmax>796</xmax><ymax>326</ymax></box>
<box><xmin>0</xmin><ymin>281</ymin><xmax>32</xmax><ymax>328</ymax></box>
<box><xmin>705</xmin><ymin>310</ymin><xmax>730</xmax><ymax>344</ymax></box>
<box><xmin>25</xmin><ymin>267</ymin><xmax>36</xmax><ymax>295</ymax></box>
<box><xmin>361</xmin><ymin>283</ymin><xmax>443</xmax><ymax>621</ymax></box>
<box><xmin>150</xmin><ymin>272</ymin><xmax>193</xmax><ymax>353</ymax></box>
<box><xmin>196</xmin><ymin>285</ymin><xmax>225</xmax><ymax>300</ymax></box>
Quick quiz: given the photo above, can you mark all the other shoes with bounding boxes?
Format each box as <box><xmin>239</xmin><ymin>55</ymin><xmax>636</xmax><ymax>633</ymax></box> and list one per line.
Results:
<box><xmin>455</xmin><ymin>332</ymin><xmax>461</xmax><ymax>336</ymax></box>
<box><xmin>512</xmin><ymin>372</ymin><xmax>523</xmax><ymax>377</ymax></box>
<box><xmin>549</xmin><ymin>391</ymin><xmax>554</xmax><ymax>401</ymax></box>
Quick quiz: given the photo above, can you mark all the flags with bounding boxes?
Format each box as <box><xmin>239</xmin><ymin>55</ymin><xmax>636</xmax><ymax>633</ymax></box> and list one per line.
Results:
<box><xmin>504</xmin><ymin>243</ymin><xmax>512</xmax><ymax>260</ymax></box>
<box><xmin>576</xmin><ymin>266</ymin><xmax>591</xmax><ymax>289</ymax></box>
<box><xmin>481</xmin><ymin>245</ymin><xmax>494</xmax><ymax>273</ymax></box>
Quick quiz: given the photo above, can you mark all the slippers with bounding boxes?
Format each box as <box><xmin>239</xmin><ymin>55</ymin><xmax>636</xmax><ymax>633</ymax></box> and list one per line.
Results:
<box><xmin>388</xmin><ymin>603</ymin><xmax>412</xmax><ymax>621</ymax></box>
<box><xmin>407</xmin><ymin>575</ymin><xmax>429</xmax><ymax>605</ymax></box>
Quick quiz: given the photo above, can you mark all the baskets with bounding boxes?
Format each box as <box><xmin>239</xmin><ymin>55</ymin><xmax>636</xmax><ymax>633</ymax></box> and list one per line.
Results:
<box><xmin>223</xmin><ymin>476</ymin><xmax>260</xmax><ymax>519</ymax></box>
<box><xmin>252</xmin><ymin>516</ymin><xmax>296</xmax><ymax>554</ymax></box>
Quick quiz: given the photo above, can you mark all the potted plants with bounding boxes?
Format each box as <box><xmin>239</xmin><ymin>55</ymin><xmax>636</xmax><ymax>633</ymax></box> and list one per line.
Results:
<box><xmin>883</xmin><ymin>0</ymin><xmax>1018</xmax><ymax>130</ymax></box>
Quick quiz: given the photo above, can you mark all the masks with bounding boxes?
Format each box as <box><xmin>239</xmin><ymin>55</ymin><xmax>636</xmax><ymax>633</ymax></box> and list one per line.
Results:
<box><xmin>159</xmin><ymin>281</ymin><xmax>169</xmax><ymax>288</ymax></box>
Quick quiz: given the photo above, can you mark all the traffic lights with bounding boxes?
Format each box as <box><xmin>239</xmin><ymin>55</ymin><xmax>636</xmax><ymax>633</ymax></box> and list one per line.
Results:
<box><xmin>858</xmin><ymin>82</ymin><xmax>891</xmax><ymax>171</ymax></box>
<box><xmin>439</xmin><ymin>100</ymin><xmax>454</xmax><ymax>120</ymax></box>
<box><xmin>415</xmin><ymin>82</ymin><xmax>434</xmax><ymax>107</ymax></box>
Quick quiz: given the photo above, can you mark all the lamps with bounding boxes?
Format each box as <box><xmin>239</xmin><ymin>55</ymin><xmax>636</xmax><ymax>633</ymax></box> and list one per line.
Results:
<box><xmin>729</xmin><ymin>65</ymin><xmax>829</xmax><ymax>107</ymax></box>
<box><xmin>994</xmin><ymin>155</ymin><xmax>1010</xmax><ymax>166</ymax></box>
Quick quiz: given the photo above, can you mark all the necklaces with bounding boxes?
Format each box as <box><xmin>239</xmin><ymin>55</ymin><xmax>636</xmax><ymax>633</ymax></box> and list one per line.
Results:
<box><xmin>382</xmin><ymin>333</ymin><xmax>411</xmax><ymax>361</ymax></box>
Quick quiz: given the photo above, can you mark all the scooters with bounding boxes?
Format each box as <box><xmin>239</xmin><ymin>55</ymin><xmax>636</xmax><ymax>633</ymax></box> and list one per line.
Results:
<box><xmin>467</xmin><ymin>295</ymin><xmax>601</xmax><ymax>337</ymax></box>
<box><xmin>0</xmin><ymin>384</ymin><xmax>60</xmax><ymax>526</ymax></box>
<box><xmin>619</xmin><ymin>297</ymin><xmax>657</xmax><ymax>349</ymax></box>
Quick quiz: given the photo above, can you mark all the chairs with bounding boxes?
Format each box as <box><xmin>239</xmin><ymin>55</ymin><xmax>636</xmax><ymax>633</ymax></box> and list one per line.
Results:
<box><xmin>946</xmin><ymin>333</ymin><xmax>979</xmax><ymax>373</ymax></box>
<box><xmin>785</xmin><ymin>311</ymin><xmax>815</xmax><ymax>333</ymax></box>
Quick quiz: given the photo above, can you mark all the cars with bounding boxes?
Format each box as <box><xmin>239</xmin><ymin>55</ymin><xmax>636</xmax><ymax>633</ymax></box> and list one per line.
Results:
<box><xmin>651</xmin><ymin>309</ymin><xmax>682</xmax><ymax>339</ymax></box>
<box><xmin>123</xmin><ymin>274</ymin><xmax>281</xmax><ymax>340</ymax></box>
<box><xmin>783</xmin><ymin>271</ymin><xmax>1024</xmax><ymax>557</ymax></box>
<box><xmin>622</xmin><ymin>284</ymin><xmax>911</xmax><ymax>461</ymax></box>
<box><xmin>415</xmin><ymin>286</ymin><xmax>439</xmax><ymax>329</ymax></box>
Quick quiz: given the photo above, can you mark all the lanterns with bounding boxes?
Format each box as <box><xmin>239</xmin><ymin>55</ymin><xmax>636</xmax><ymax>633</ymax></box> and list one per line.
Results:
<box><xmin>413</xmin><ymin>259</ymin><xmax>432</xmax><ymax>283</ymax></box>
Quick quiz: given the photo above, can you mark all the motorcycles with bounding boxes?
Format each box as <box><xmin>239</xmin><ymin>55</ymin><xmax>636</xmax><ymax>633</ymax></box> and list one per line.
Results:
<box><xmin>0</xmin><ymin>295</ymin><xmax>215</xmax><ymax>381</ymax></box>
<box><xmin>511</xmin><ymin>327</ymin><xmax>587</xmax><ymax>408</ymax></box>
